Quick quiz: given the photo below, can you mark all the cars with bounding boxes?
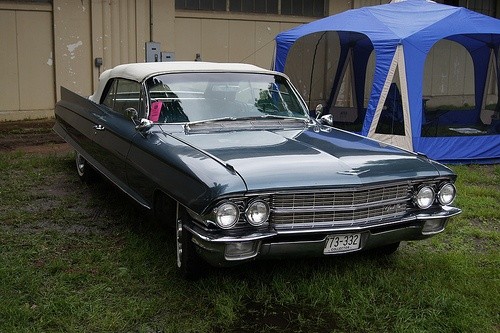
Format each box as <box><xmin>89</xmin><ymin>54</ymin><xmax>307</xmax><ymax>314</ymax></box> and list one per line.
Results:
<box><xmin>52</xmin><ymin>62</ymin><xmax>460</xmax><ymax>278</ymax></box>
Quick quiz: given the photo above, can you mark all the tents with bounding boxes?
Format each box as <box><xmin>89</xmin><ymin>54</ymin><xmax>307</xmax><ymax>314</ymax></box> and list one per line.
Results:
<box><xmin>271</xmin><ymin>0</ymin><xmax>500</xmax><ymax>167</ymax></box>
